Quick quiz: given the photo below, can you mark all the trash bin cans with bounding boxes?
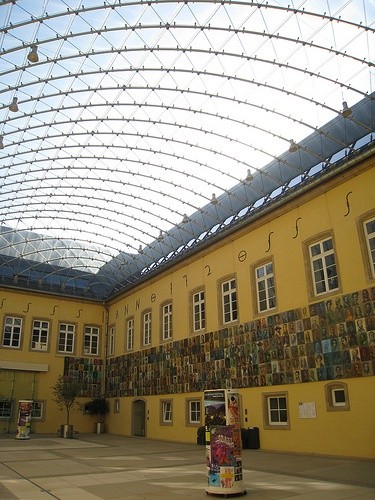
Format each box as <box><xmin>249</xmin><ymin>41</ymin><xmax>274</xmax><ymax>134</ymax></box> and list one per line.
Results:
<box><xmin>95</xmin><ymin>421</ymin><xmax>105</xmax><ymax>434</ymax></box>
<box><xmin>61</xmin><ymin>424</ymin><xmax>73</xmax><ymax>439</ymax></box>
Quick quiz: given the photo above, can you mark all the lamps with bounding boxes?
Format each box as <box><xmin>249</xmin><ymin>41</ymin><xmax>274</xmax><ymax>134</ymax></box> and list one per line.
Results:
<box><xmin>138</xmin><ymin>245</ymin><xmax>143</xmax><ymax>253</ymax></box>
<box><xmin>210</xmin><ymin>193</ymin><xmax>219</xmax><ymax>205</ymax></box>
<box><xmin>157</xmin><ymin>230</ymin><xmax>164</xmax><ymax>239</ymax></box>
<box><xmin>288</xmin><ymin>139</ymin><xmax>298</xmax><ymax>152</ymax></box>
<box><xmin>246</xmin><ymin>169</ymin><xmax>254</xmax><ymax>182</ymax></box>
<box><xmin>27</xmin><ymin>44</ymin><xmax>39</xmax><ymax>63</ymax></box>
<box><xmin>0</xmin><ymin>134</ymin><xmax>5</xmax><ymax>150</ymax></box>
<box><xmin>182</xmin><ymin>214</ymin><xmax>189</xmax><ymax>224</ymax></box>
<box><xmin>9</xmin><ymin>97</ymin><xmax>19</xmax><ymax>112</ymax></box>
<box><xmin>341</xmin><ymin>101</ymin><xmax>352</xmax><ymax>118</ymax></box>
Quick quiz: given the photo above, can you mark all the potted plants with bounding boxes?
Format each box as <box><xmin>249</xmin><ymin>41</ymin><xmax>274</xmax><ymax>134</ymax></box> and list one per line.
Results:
<box><xmin>49</xmin><ymin>372</ymin><xmax>87</xmax><ymax>439</ymax></box>
<box><xmin>83</xmin><ymin>397</ymin><xmax>110</xmax><ymax>436</ymax></box>
